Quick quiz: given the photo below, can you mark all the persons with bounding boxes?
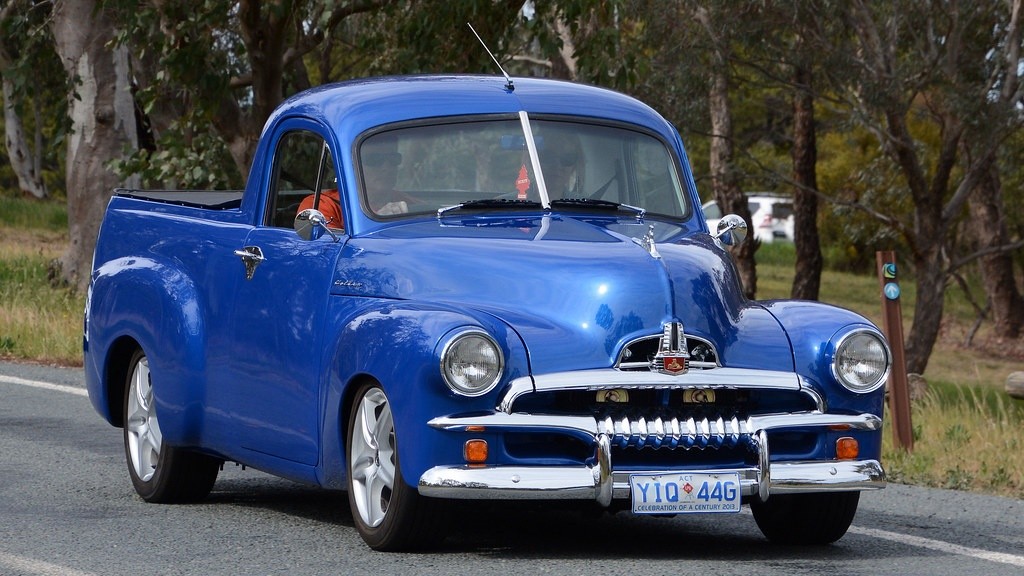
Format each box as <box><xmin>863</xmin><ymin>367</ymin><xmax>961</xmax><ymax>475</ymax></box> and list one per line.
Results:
<box><xmin>295</xmin><ymin>132</ymin><xmax>424</xmax><ymax>232</ymax></box>
<box><xmin>492</xmin><ymin>123</ymin><xmax>588</xmax><ymax>201</ymax></box>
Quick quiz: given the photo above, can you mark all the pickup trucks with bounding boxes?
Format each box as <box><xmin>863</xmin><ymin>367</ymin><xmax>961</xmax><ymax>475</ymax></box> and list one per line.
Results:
<box><xmin>82</xmin><ymin>72</ymin><xmax>896</xmax><ymax>554</ymax></box>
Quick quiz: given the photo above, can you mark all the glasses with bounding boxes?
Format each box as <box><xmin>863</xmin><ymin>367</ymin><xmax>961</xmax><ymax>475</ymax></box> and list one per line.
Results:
<box><xmin>542</xmin><ymin>151</ymin><xmax>579</xmax><ymax>166</ymax></box>
<box><xmin>361</xmin><ymin>152</ymin><xmax>403</xmax><ymax>168</ymax></box>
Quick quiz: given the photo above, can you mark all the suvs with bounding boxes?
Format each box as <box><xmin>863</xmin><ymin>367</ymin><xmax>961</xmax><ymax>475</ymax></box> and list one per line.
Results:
<box><xmin>701</xmin><ymin>192</ymin><xmax>794</xmax><ymax>244</ymax></box>
